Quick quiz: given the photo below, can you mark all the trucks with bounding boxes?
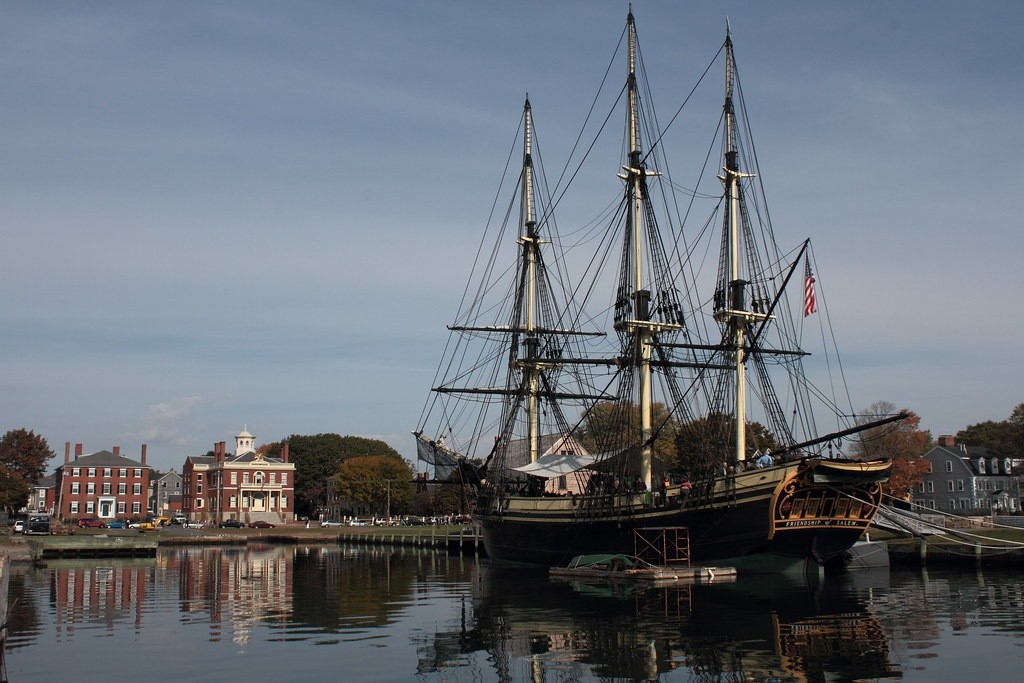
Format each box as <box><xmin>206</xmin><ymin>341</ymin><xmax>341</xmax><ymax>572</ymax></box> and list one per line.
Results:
<box><xmin>25</xmin><ymin>513</ymin><xmax>51</xmax><ymax>536</ymax></box>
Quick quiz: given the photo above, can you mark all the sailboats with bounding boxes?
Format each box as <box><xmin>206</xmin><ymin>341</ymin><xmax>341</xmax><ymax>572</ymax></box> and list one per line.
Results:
<box><xmin>412</xmin><ymin>4</ymin><xmax>910</xmax><ymax>572</ymax></box>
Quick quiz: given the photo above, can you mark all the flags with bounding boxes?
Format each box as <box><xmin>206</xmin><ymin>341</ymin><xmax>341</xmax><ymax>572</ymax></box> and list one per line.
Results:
<box><xmin>805</xmin><ymin>254</ymin><xmax>816</xmax><ymax>317</ymax></box>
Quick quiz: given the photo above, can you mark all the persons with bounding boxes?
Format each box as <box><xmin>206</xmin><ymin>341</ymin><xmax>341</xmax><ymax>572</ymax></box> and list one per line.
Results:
<box><xmin>528</xmin><ymin>476</ymin><xmax>543</xmax><ymax>497</ymax></box>
<box><xmin>305</xmin><ymin>517</ymin><xmax>311</xmax><ymax>529</ymax></box>
<box><xmin>594</xmin><ymin>474</ymin><xmax>693</xmax><ymax>513</ymax></box>
<box><xmin>719</xmin><ymin>450</ymin><xmax>781</xmax><ymax>476</ymax></box>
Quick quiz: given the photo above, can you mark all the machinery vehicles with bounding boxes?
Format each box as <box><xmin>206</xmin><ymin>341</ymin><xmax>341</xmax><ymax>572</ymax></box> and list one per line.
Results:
<box><xmin>139</xmin><ymin>516</ymin><xmax>170</xmax><ymax>533</ymax></box>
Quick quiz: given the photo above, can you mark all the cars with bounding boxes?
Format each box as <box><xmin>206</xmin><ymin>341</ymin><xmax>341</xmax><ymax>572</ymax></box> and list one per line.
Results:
<box><xmin>183</xmin><ymin>519</ymin><xmax>203</xmax><ymax>528</ymax></box>
<box><xmin>321</xmin><ymin>520</ymin><xmax>343</xmax><ymax>528</ymax></box>
<box><xmin>348</xmin><ymin>520</ymin><xmax>368</xmax><ymax>527</ymax></box>
<box><xmin>128</xmin><ymin>521</ymin><xmax>141</xmax><ymax>528</ymax></box>
<box><xmin>13</xmin><ymin>520</ymin><xmax>23</xmax><ymax>531</ymax></box>
<box><xmin>249</xmin><ymin>521</ymin><xmax>275</xmax><ymax>529</ymax></box>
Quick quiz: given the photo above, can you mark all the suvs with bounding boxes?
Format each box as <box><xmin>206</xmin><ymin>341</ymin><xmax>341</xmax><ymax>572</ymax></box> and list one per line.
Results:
<box><xmin>173</xmin><ymin>514</ymin><xmax>186</xmax><ymax>521</ymax></box>
<box><xmin>78</xmin><ymin>518</ymin><xmax>105</xmax><ymax>529</ymax></box>
<box><xmin>375</xmin><ymin>517</ymin><xmax>397</xmax><ymax>526</ymax></box>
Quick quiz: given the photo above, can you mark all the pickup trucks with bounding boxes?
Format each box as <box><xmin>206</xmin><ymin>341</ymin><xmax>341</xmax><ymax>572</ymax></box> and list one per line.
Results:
<box><xmin>108</xmin><ymin>518</ymin><xmax>127</xmax><ymax>530</ymax></box>
<box><xmin>219</xmin><ymin>518</ymin><xmax>245</xmax><ymax>529</ymax></box>
<box><xmin>403</xmin><ymin>517</ymin><xmax>424</xmax><ymax>526</ymax></box>
